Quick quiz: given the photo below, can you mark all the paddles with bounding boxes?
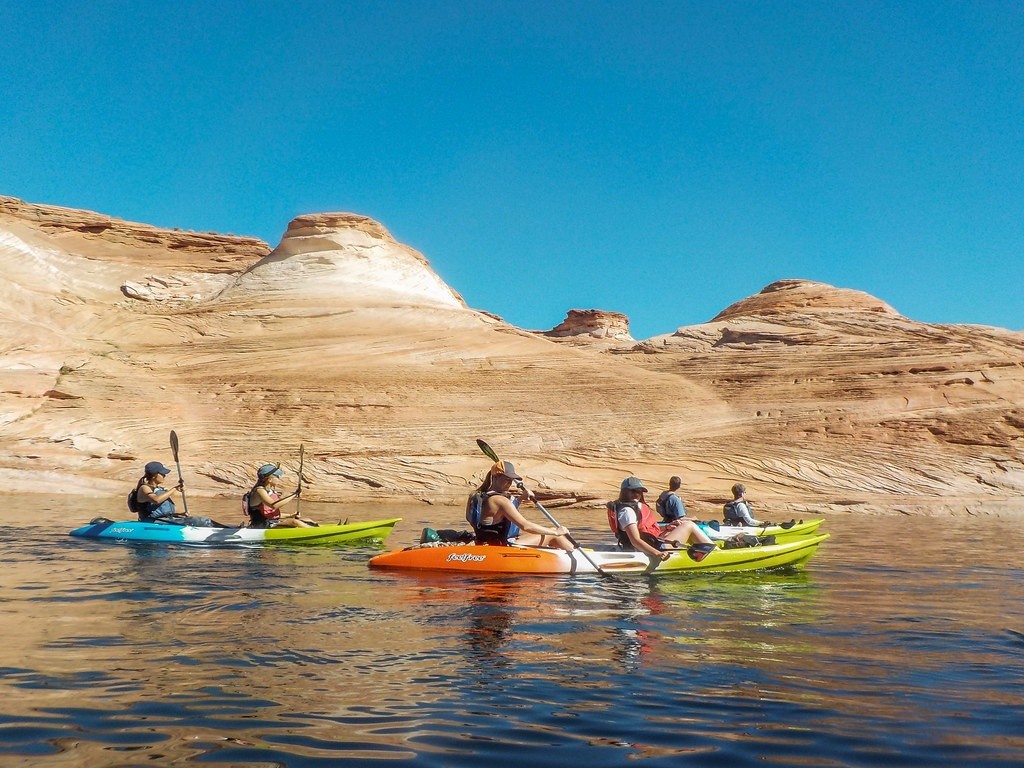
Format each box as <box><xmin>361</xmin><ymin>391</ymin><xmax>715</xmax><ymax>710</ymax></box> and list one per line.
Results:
<box><xmin>656</xmin><ymin>538</ymin><xmax>717</xmax><ymax>562</ymax></box>
<box><xmin>765</xmin><ymin>522</ymin><xmax>796</xmax><ymax>530</ymax></box>
<box><xmin>694</xmin><ymin>520</ymin><xmax>720</xmax><ymax>532</ymax></box>
<box><xmin>296</xmin><ymin>443</ymin><xmax>304</xmax><ymax>514</ymax></box>
<box><xmin>476</xmin><ymin>439</ymin><xmax>612</xmax><ymax>577</ymax></box>
<box><xmin>169</xmin><ymin>430</ymin><xmax>188</xmax><ymax>513</ymax></box>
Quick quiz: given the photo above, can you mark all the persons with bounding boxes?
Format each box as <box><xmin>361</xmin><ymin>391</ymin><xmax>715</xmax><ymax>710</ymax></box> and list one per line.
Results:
<box><xmin>135</xmin><ymin>461</ymin><xmax>244</xmax><ymax>529</ymax></box>
<box><xmin>727</xmin><ymin>482</ymin><xmax>803</xmax><ymax>527</ymax></box>
<box><xmin>614</xmin><ymin>477</ymin><xmax>721</xmax><ymax>559</ymax></box>
<box><xmin>248</xmin><ymin>463</ymin><xmax>350</xmax><ymax>529</ymax></box>
<box><xmin>474</xmin><ymin>461</ymin><xmax>575</xmax><ymax>551</ymax></box>
<box><xmin>659</xmin><ymin>475</ymin><xmax>699</xmax><ymax>523</ymax></box>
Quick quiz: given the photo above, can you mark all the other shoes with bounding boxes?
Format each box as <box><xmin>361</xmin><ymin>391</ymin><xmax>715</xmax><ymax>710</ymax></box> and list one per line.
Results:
<box><xmin>239</xmin><ymin>521</ymin><xmax>245</xmax><ymax>528</ymax></box>
<box><xmin>343</xmin><ymin>517</ymin><xmax>349</xmax><ymax>525</ymax></box>
<box><xmin>337</xmin><ymin>518</ymin><xmax>342</xmax><ymax>525</ymax></box>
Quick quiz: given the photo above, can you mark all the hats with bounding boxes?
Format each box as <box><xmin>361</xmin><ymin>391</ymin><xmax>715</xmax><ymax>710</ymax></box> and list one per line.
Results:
<box><xmin>621</xmin><ymin>477</ymin><xmax>648</xmax><ymax>492</ymax></box>
<box><xmin>257</xmin><ymin>464</ymin><xmax>284</xmax><ymax>478</ymax></box>
<box><xmin>491</xmin><ymin>461</ymin><xmax>523</xmax><ymax>481</ymax></box>
<box><xmin>145</xmin><ymin>462</ymin><xmax>169</xmax><ymax>476</ymax></box>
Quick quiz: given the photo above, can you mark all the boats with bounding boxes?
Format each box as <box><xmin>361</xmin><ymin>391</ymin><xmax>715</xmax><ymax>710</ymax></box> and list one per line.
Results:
<box><xmin>67</xmin><ymin>517</ymin><xmax>403</xmax><ymax>546</ymax></box>
<box><xmin>690</xmin><ymin>518</ymin><xmax>827</xmax><ymax>544</ymax></box>
<box><xmin>366</xmin><ymin>532</ymin><xmax>831</xmax><ymax>575</ymax></box>
